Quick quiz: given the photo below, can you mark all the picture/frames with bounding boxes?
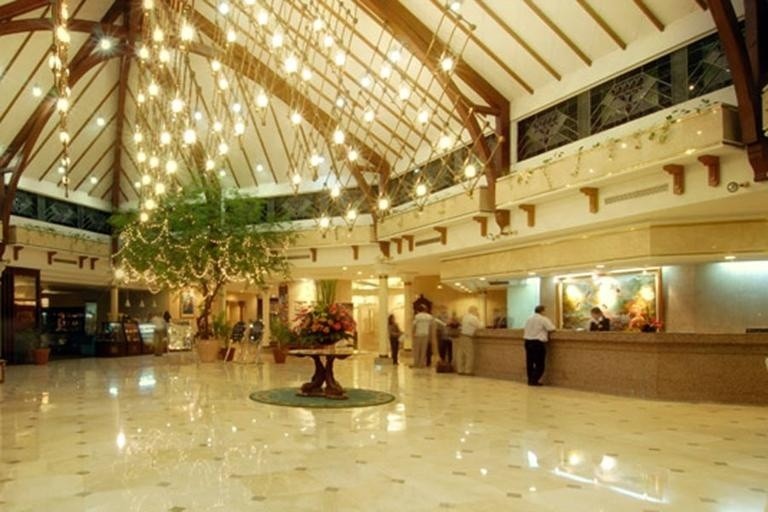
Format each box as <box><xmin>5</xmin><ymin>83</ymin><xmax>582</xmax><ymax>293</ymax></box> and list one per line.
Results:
<box><xmin>556</xmin><ymin>268</ymin><xmax>664</xmax><ymax>332</ymax></box>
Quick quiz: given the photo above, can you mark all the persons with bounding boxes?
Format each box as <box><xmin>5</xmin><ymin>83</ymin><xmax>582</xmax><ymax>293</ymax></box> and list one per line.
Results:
<box><xmin>388</xmin><ymin>314</ymin><xmax>401</xmax><ymax>365</ymax></box>
<box><xmin>493</xmin><ymin>305</ymin><xmax>514</xmax><ymax>328</ymax></box>
<box><xmin>589</xmin><ymin>307</ymin><xmax>610</xmax><ymax>331</ymax></box>
<box><xmin>522</xmin><ymin>304</ymin><xmax>557</xmax><ymax>385</ymax></box>
<box><xmin>456</xmin><ymin>304</ymin><xmax>485</xmax><ymax>375</ymax></box>
<box><xmin>408</xmin><ymin>303</ymin><xmax>446</xmax><ymax>368</ymax></box>
<box><xmin>436</xmin><ymin>305</ymin><xmax>448</xmax><ymax>363</ymax></box>
<box><xmin>439</xmin><ymin>309</ymin><xmax>462</xmax><ymax>363</ymax></box>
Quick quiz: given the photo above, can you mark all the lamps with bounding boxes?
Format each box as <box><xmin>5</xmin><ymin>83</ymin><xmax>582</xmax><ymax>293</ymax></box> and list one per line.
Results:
<box><xmin>728</xmin><ymin>182</ymin><xmax>750</xmax><ymax>192</ymax></box>
<box><xmin>33</xmin><ymin>0</ymin><xmax>506</xmax><ymax>239</ymax></box>
<box><xmin>488</xmin><ymin>230</ymin><xmax>512</xmax><ymax>242</ymax></box>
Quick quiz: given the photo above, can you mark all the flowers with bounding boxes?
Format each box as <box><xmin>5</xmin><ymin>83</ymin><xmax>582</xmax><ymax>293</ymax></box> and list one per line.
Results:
<box><xmin>289</xmin><ymin>280</ymin><xmax>357</xmax><ymax>346</ymax></box>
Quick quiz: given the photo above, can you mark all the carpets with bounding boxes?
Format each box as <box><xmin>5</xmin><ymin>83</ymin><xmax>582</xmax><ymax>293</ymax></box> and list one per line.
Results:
<box><xmin>250</xmin><ymin>387</ymin><xmax>395</xmax><ymax>408</ymax></box>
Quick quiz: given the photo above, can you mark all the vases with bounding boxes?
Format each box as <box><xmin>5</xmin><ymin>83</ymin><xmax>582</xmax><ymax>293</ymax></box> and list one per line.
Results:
<box><xmin>315</xmin><ymin>338</ymin><xmax>340</xmax><ymax>346</ymax></box>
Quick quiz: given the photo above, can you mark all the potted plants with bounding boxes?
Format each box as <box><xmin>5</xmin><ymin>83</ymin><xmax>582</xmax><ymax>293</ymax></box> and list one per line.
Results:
<box><xmin>269</xmin><ymin>314</ymin><xmax>288</xmax><ymax>364</ymax></box>
<box><xmin>31</xmin><ymin>333</ymin><xmax>51</xmax><ymax>365</ymax></box>
<box><xmin>219</xmin><ymin>320</ymin><xmax>235</xmax><ymax>361</ymax></box>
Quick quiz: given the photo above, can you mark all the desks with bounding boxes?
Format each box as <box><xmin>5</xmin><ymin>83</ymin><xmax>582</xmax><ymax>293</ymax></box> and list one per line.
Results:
<box><xmin>289</xmin><ymin>350</ymin><xmax>352</xmax><ymax>400</ymax></box>
